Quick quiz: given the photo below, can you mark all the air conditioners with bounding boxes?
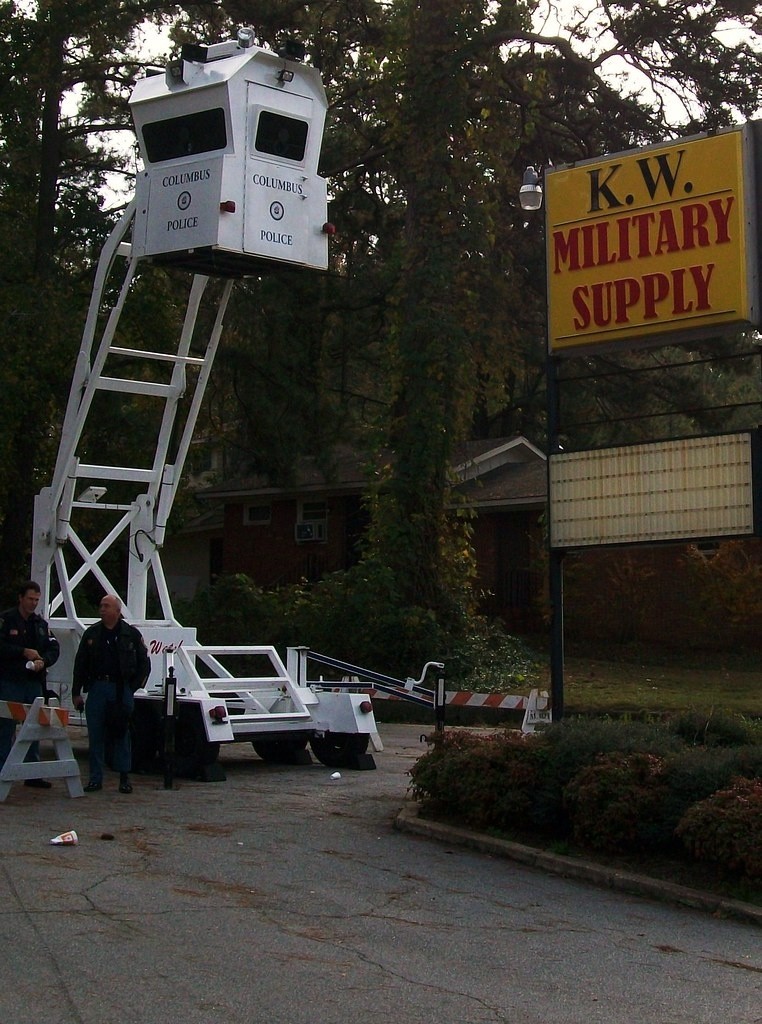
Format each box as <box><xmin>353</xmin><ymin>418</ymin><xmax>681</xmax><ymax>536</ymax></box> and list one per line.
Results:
<box><xmin>294</xmin><ymin>521</ymin><xmax>325</xmax><ymax>542</ymax></box>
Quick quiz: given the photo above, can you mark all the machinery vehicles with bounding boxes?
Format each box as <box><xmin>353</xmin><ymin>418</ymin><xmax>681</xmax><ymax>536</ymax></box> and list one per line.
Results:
<box><xmin>27</xmin><ymin>24</ymin><xmax>385</xmax><ymax>778</ymax></box>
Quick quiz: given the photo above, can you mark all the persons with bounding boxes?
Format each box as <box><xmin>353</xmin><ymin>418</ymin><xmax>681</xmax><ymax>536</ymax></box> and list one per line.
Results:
<box><xmin>72</xmin><ymin>596</ymin><xmax>151</xmax><ymax>793</ymax></box>
<box><xmin>0</xmin><ymin>581</ymin><xmax>59</xmax><ymax>789</ymax></box>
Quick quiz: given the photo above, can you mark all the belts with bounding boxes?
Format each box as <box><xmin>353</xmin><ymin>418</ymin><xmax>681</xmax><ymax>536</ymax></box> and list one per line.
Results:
<box><xmin>98</xmin><ymin>673</ymin><xmax>117</xmax><ymax>683</ymax></box>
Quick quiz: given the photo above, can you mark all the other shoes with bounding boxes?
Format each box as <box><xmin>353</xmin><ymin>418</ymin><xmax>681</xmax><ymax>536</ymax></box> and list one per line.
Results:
<box><xmin>84</xmin><ymin>782</ymin><xmax>102</xmax><ymax>792</ymax></box>
<box><xmin>119</xmin><ymin>783</ymin><xmax>133</xmax><ymax>793</ymax></box>
<box><xmin>24</xmin><ymin>779</ymin><xmax>51</xmax><ymax>788</ymax></box>
